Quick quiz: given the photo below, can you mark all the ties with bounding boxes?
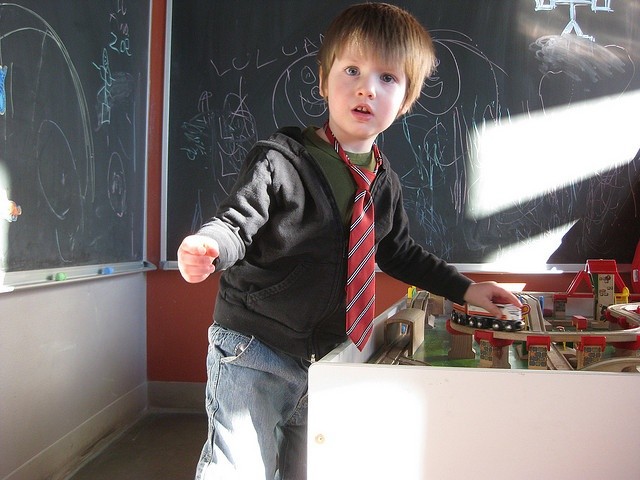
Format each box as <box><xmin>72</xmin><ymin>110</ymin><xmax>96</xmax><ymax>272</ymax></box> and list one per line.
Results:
<box><xmin>323</xmin><ymin>119</ymin><xmax>383</xmax><ymax>352</ymax></box>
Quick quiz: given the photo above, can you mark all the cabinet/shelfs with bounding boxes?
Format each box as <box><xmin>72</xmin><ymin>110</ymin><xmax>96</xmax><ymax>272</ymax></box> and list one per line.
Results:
<box><xmin>307</xmin><ymin>285</ymin><xmax>640</xmax><ymax>479</ymax></box>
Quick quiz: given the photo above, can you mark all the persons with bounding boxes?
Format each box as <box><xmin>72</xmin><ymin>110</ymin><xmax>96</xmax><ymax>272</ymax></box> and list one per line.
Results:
<box><xmin>177</xmin><ymin>0</ymin><xmax>525</xmax><ymax>480</ymax></box>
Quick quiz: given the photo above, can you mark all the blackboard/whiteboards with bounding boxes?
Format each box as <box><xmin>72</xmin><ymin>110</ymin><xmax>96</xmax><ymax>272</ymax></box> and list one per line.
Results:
<box><xmin>1</xmin><ymin>2</ymin><xmax>152</xmax><ymax>292</ymax></box>
<box><xmin>159</xmin><ymin>0</ymin><xmax>640</xmax><ymax>273</ymax></box>
